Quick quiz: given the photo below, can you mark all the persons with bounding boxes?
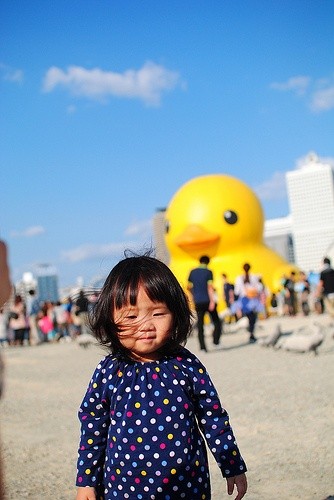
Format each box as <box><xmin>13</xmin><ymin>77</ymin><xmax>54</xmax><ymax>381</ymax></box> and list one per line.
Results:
<box><xmin>0</xmin><ymin>241</ymin><xmax>100</xmax><ymax>397</ymax></box>
<box><xmin>188</xmin><ymin>257</ymin><xmax>222</xmax><ymax>352</ymax></box>
<box><xmin>209</xmin><ymin>258</ymin><xmax>334</xmax><ymax>342</ymax></box>
<box><xmin>76</xmin><ymin>255</ymin><xmax>248</xmax><ymax>500</ymax></box>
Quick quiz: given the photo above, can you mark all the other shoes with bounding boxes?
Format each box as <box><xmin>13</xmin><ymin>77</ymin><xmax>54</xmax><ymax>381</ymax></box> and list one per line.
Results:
<box><xmin>201</xmin><ymin>347</ymin><xmax>208</xmax><ymax>353</ymax></box>
<box><xmin>250</xmin><ymin>335</ymin><xmax>257</xmax><ymax>343</ymax></box>
<box><xmin>215</xmin><ymin>344</ymin><xmax>222</xmax><ymax>350</ymax></box>
